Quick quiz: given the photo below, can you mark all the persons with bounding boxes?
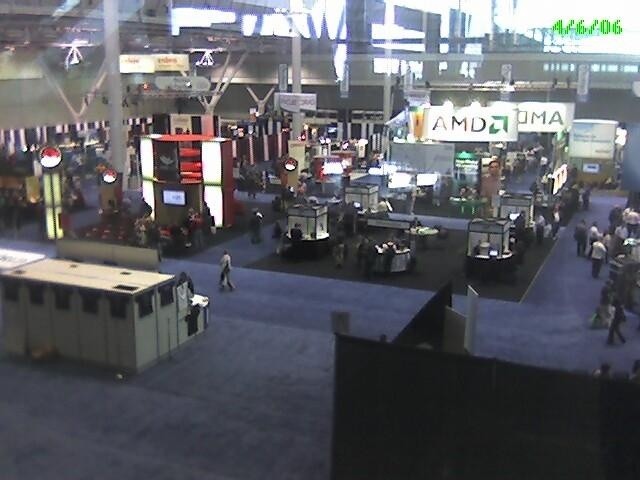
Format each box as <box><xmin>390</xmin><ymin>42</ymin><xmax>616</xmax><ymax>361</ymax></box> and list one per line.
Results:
<box><xmin>473</xmin><ymin>145</ymin><xmax>640</xmax><ymax>382</ymax></box>
<box><xmin>62</xmin><ymin>155</ymin><xmax>421</xmax><ymax>312</ymax></box>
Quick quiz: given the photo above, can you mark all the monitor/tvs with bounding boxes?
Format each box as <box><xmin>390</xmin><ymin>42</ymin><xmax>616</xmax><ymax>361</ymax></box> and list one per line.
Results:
<box><xmin>162</xmin><ymin>190</ymin><xmax>186</xmax><ymax>206</ymax></box>
